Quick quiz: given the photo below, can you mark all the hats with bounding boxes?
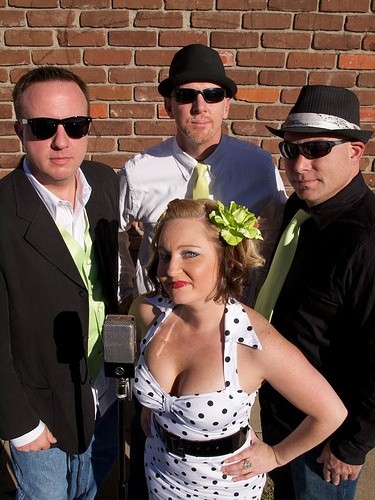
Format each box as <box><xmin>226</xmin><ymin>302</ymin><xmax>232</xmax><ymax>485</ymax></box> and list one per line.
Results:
<box><xmin>158</xmin><ymin>44</ymin><xmax>237</xmax><ymax>100</ymax></box>
<box><xmin>264</xmin><ymin>85</ymin><xmax>375</xmax><ymax>145</ymax></box>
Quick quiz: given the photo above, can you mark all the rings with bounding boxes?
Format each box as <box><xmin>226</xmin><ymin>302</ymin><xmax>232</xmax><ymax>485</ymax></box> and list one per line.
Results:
<box><xmin>243</xmin><ymin>459</ymin><xmax>251</xmax><ymax>469</ymax></box>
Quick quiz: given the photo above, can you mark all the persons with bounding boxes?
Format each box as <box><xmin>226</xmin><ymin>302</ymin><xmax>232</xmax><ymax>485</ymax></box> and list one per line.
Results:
<box><xmin>118</xmin><ymin>44</ymin><xmax>289</xmax><ymax>308</ymax></box>
<box><xmin>0</xmin><ymin>67</ymin><xmax>121</xmax><ymax>500</ymax></box>
<box><xmin>127</xmin><ymin>199</ymin><xmax>348</xmax><ymax>500</ymax></box>
<box><xmin>254</xmin><ymin>85</ymin><xmax>375</xmax><ymax>500</ymax></box>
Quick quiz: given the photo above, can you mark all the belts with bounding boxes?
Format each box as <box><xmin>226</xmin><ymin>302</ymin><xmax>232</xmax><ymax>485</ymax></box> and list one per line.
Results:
<box><xmin>153</xmin><ymin>415</ymin><xmax>248</xmax><ymax>457</ymax></box>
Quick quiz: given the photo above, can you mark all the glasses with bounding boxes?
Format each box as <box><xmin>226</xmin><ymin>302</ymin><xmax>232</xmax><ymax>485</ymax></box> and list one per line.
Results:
<box><xmin>171</xmin><ymin>87</ymin><xmax>229</xmax><ymax>104</ymax></box>
<box><xmin>20</xmin><ymin>115</ymin><xmax>94</xmax><ymax>141</ymax></box>
<box><xmin>278</xmin><ymin>139</ymin><xmax>354</xmax><ymax>160</ymax></box>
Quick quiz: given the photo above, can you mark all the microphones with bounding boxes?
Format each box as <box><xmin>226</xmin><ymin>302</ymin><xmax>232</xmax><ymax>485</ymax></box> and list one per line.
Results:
<box><xmin>102</xmin><ymin>315</ymin><xmax>137</xmax><ymax>378</ymax></box>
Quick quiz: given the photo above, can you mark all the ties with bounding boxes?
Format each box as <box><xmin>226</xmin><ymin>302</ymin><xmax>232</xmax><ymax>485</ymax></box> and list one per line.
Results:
<box><xmin>254</xmin><ymin>209</ymin><xmax>311</xmax><ymax>322</ymax></box>
<box><xmin>191</xmin><ymin>163</ymin><xmax>210</xmax><ymax>200</ymax></box>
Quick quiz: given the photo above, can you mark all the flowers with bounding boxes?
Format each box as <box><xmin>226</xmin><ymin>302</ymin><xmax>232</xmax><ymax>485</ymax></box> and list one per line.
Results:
<box><xmin>209</xmin><ymin>200</ymin><xmax>265</xmax><ymax>246</ymax></box>
